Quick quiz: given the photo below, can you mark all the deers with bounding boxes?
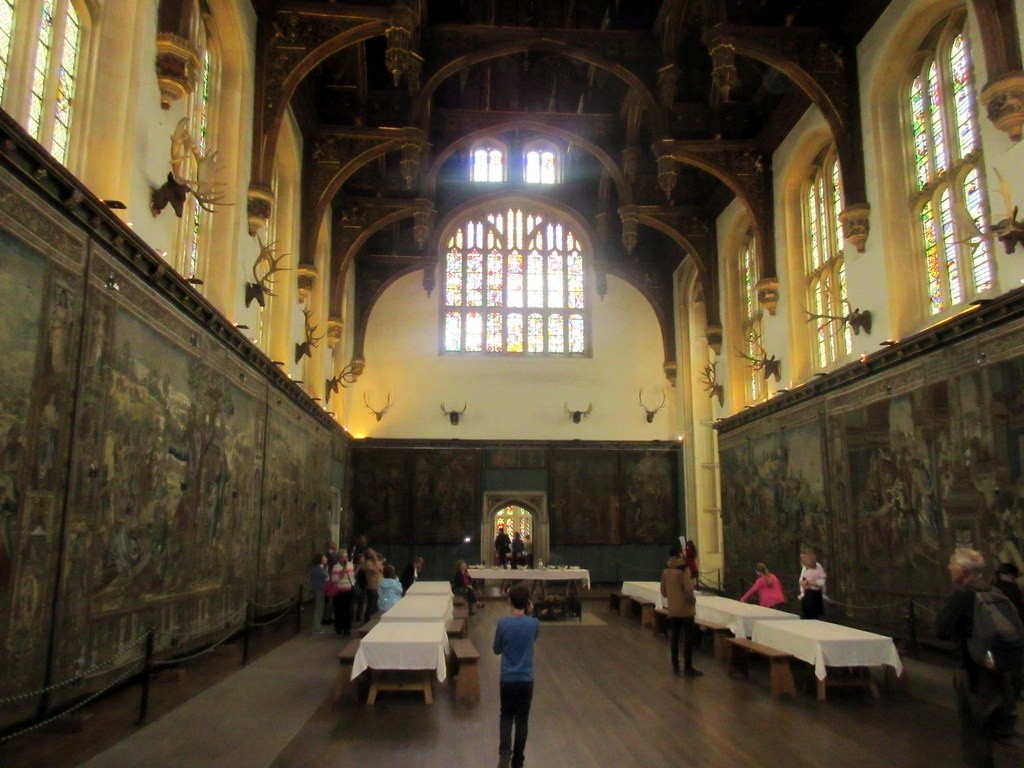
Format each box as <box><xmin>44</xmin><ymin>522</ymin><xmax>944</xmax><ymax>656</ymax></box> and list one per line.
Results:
<box><xmin>800</xmin><ymin>273</ymin><xmax>872</xmax><ymax>343</ymax></box>
<box><xmin>955</xmin><ymin>169</ymin><xmax>1024</xmax><ymax>255</ymax></box>
<box><xmin>245</xmin><ymin>235</ymin><xmax>291</xmax><ymax>309</ymax></box>
<box><xmin>150</xmin><ymin>117</ymin><xmax>236</xmax><ymax>218</ymax></box>
<box><xmin>699</xmin><ymin>359</ymin><xmax>723</xmax><ymax>407</ymax></box>
<box><xmin>563</xmin><ymin>402</ymin><xmax>593</xmax><ymax>424</ymax></box>
<box><xmin>295</xmin><ymin>308</ymin><xmax>324</xmax><ymax>364</ymax></box>
<box><xmin>363</xmin><ymin>393</ymin><xmax>391</xmax><ymax>422</ymax></box>
<box><xmin>326</xmin><ymin>364</ymin><xmax>359</xmax><ymax>404</ymax></box>
<box><xmin>639</xmin><ymin>388</ymin><xmax>665</xmax><ymax>423</ymax></box>
<box><xmin>733</xmin><ymin>329</ymin><xmax>781</xmax><ymax>382</ymax></box>
<box><xmin>440</xmin><ymin>400</ymin><xmax>467</xmax><ymax>425</ymax></box>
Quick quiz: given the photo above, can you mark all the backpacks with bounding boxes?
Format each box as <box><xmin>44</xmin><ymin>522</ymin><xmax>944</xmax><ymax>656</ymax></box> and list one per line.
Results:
<box><xmin>959</xmin><ymin>584</ymin><xmax>1024</xmax><ymax>674</ymax></box>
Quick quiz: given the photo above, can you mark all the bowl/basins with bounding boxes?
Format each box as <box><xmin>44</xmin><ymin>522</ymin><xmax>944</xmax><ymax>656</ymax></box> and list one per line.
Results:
<box><xmin>517</xmin><ymin>565</ymin><xmax>528</xmax><ymax>570</ymax></box>
<box><xmin>548</xmin><ymin>565</ymin><xmax>580</xmax><ymax>569</ymax></box>
<box><xmin>469</xmin><ymin>565</ymin><xmax>500</xmax><ymax>570</ymax></box>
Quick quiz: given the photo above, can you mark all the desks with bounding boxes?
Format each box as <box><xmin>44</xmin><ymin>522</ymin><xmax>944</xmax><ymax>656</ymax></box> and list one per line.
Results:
<box><xmin>350</xmin><ymin>621</ymin><xmax>451</xmax><ymax>704</ymax></box>
<box><xmin>750</xmin><ymin>619</ymin><xmax>903</xmax><ymax>701</ymax></box>
<box><xmin>621</xmin><ymin>580</ymin><xmax>702</xmax><ymax>610</ymax></box>
<box><xmin>405</xmin><ymin>581</ymin><xmax>454</xmax><ymax>597</ymax></box>
<box><xmin>693</xmin><ymin>596</ymin><xmax>799</xmax><ymax>640</ymax></box>
<box><xmin>381</xmin><ymin>596</ymin><xmax>455</xmax><ymax>622</ymax></box>
<box><xmin>468</xmin><ymin>567</ymin><xmax>590</xmax><ymax>596</ymax></box>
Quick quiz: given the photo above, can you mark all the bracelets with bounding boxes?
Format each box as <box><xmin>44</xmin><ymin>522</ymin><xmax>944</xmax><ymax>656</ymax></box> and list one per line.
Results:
<box><xmin>806</xmin><ymin>580</ymin><xmax>808</xmax><ymax>585</ymax></box>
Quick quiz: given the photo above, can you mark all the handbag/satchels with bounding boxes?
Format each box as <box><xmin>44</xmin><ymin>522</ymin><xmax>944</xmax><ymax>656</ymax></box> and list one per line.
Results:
<box><xmin>350</xmin><ymin>583</ymin><xmax>362</xmax><ymax>600</ymax></box>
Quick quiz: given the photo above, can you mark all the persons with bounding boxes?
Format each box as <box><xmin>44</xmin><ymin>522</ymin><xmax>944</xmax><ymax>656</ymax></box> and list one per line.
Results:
<box><xmin>400</xmin><ymin>556</ymin><xmax>424</xmax><ymax>591</ymax></box>
<box><xmin>741</xmin><ymin>562</ymin><xmax>786</xmax><ymax>611</ymax></box>
<box><xmin>495</xmin><ymin>528</ymin><xmax>510</xmax><ymax>565</ymax></box>
<box><xmin>659</xmin><ymin>545</ymin><xmax>704</xmax><ymax>677</ymax></box>
<box><xmin>511</xmin><ymin>532</ymin><xmax>522</xmax><ymax>569</ymax></box>
<box><xmin>994</xmin><ymin>563</ymin><xmax>1024</xmax><ymax>701</ymax></box>
<box><xmin>798</xmin><ymin>549</ymin><xmax>826</xmax><ymax>619</ymax></box>
<box><xmin>377</xmin><ymin>565</ymin><xmax>403</xmax><ymax>611</ymax></box>
<box><xmin>936</xmin><ymin>548</ymin><xmax>1013</xmax><ymax>768</ymax></box>
<box><xmin>523</xmin><ymin>535</ymin><xmax>533</xmax><ymax>569</ymax></box>
<box><xmin>492</xmin><ymin>586</ymin><xmax>540</xmax><ymax>768</ymax></box>
<box><xmin>453</xmin><ymin>560</ymin><xmax>485</xmax><ymax>616</ymax></box>
<box><xmin>312</xmin><ymin>534</ymin><xmax>384</xmax><ymax>640</ymax></box>
<box><xmin>683</xmin><ymin>540</ymin><xmax>699</xmax><ymax>580</ymax></box>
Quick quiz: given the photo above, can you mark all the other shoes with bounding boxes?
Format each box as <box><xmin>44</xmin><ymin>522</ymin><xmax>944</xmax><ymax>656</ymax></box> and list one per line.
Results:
<box><xmin>343</xmin><ymin>629</ymin><xmax>352</xmax><ymax>636</ymax></box>
<box><xmin>683</xmin><ymin>669</ymin><xmax>704</xmax><ymax>677</ymax></box>
<box><xmin>497</xmin><ymin>751</ymin><xmax>511</xmax><ymax>768</ymax></box>
<box><xmin>322</xmin><ymin>618</ymin><xmax>334</xmax><ymax>624</ymax></box>
<box><xmin>337</xmin><ymin>629</ymin><xmax>343</xmax><ymax>634</ymax></box>
<box><xmin>311</xmin><ymin>628</ymin><xmax>325</xmax><ymax>634</ymax></box>
<box><xmin>673</xmin><ymin>662</ymin><xmax>680</xmax><ymax>674</ymax></box>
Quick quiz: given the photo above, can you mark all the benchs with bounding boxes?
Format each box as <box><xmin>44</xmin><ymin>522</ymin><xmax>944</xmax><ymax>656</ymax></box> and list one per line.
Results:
<box><xmin>610</xmin><ymin>591</ymin><xmax>797</xmax><ymax>696</ymax></box>
<box><xmin>447</xmin><ymin>595</ymin><xmax>480</xmax><ymax>705</ymax></box>
<box><xmin>335</xmin><ymin>609</ymin><xmax>388</xmax><ymax>701</ymax></box>
